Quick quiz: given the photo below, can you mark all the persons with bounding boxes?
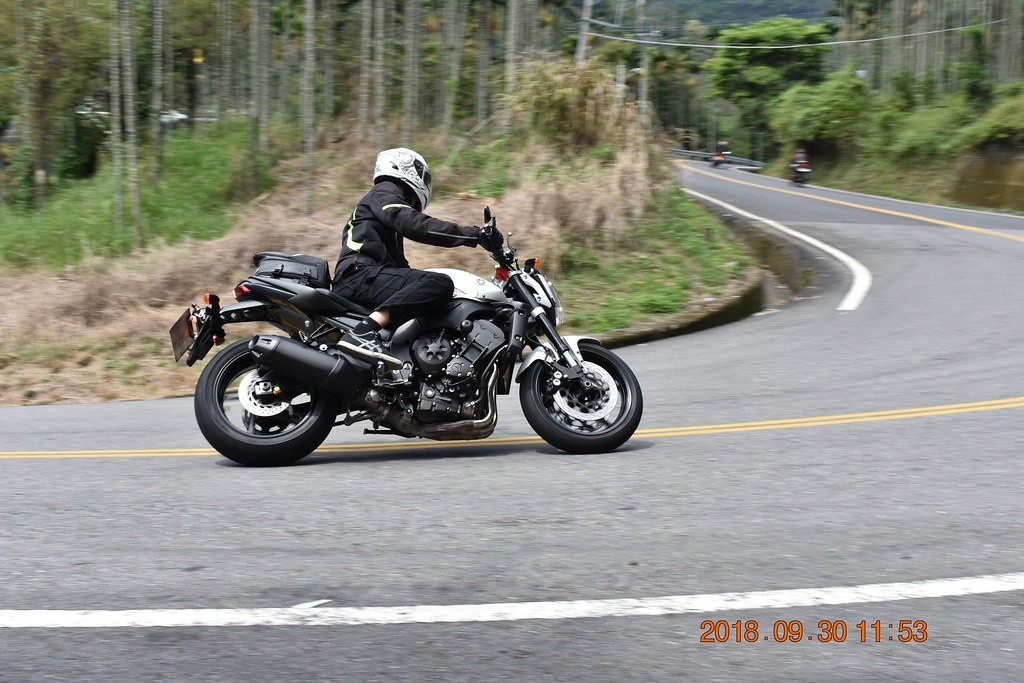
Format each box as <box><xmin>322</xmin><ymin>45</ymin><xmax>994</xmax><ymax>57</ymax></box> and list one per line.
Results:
<box><xmin>332</xmin><ymin>144</ymin><xmax>503</xmax><ymax>372</ymax></box>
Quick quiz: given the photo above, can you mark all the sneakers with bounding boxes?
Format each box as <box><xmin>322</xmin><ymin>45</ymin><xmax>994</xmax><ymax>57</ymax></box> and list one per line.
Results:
<box><xmin>338</xmin><ymin>330</ymin><xmax>404</xmax><ymax>372</ymax></box>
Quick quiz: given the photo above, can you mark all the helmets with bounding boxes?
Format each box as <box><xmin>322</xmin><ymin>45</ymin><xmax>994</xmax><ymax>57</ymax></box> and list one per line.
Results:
<box><xmin>372</xmin><ymin>146</ymin><xmax>431</xmax><ymax>213</ymax></box>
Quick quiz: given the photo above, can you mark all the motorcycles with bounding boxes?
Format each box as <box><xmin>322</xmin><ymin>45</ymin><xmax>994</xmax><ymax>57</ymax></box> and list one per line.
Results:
<box><xmin>789</xmin><ymin>160</ymin><xmax>812</xmax><ymax>184</ymax></box>
<box><xmin>169</xmin><ymin>206</ymin><xmax>643</xmax><ymax>468</ymax></box>
<box><xmin>713</xmin><ymin>147</ymin><xmax>732</xmax><ymax>166</ymax></box>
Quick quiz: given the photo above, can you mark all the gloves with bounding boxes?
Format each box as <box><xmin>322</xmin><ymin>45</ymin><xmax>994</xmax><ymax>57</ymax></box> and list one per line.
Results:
<box><xmin>479</xmin><ymin>224</ymin><xmax>503</xmax><ymax>252</ymax></box>
